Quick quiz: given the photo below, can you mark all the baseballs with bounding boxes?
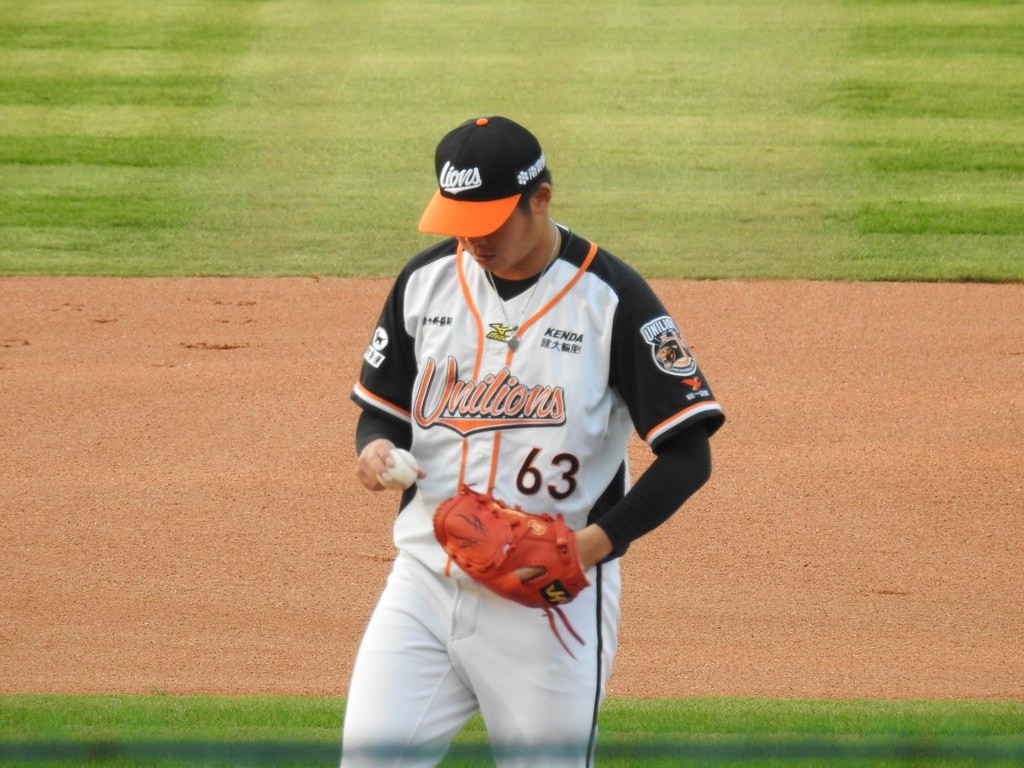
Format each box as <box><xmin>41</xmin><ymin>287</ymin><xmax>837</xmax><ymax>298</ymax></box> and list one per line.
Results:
<box><xmin>376</xmin><ymin>447</ymin><xmax>418</xmax><ymax>491</ymax></box>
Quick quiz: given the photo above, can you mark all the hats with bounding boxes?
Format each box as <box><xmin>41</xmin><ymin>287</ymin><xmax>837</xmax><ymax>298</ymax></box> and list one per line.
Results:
<box><xmin>418</xmin><ymin>115</ymin><xmax>546</xmax><ymax>238</ymax></box>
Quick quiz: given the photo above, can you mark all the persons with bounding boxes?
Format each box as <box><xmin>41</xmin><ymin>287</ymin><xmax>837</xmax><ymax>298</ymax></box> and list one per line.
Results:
<box><xmin>339</xmin><ymin>116</ymin><xmax>726</xmax><ymax>768</ymax></box>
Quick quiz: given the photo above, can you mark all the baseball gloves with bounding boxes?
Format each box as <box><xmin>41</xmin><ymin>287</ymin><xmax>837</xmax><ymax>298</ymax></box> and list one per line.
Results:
<box><xmin>432</xmin><ymin>484</ymin><xmax>592</xmax><ymax>609</ymax></box>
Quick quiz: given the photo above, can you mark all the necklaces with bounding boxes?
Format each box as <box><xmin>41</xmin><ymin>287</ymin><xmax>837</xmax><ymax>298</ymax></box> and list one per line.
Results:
<box><xmin>487</xmin><ymin>218</ymin><xmax>557</xmax><ymax>351</ymax></box>
